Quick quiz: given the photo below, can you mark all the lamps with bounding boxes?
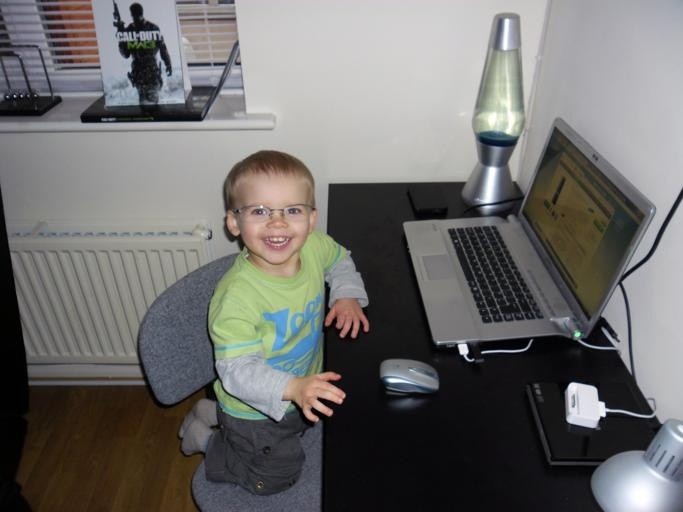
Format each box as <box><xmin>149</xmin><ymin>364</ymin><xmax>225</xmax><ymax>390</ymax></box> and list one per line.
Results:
<box><xmin>590</xmin><ymin>418</ymin><xmax>683</xmax><ymax>512</ymax></box>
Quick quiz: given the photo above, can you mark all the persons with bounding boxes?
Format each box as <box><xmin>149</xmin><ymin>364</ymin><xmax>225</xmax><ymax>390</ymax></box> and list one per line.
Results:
<box><xmin>177</xmin><ymin>151</ymin><xmax>370</xmax><ymax>495</ymax></box>
<box><xmin>118</xmin><ymin>3</ymin><xmax>172</xmax><ymax>105</ymax></box>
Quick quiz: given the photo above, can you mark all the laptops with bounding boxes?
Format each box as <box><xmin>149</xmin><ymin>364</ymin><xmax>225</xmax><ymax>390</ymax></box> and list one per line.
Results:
<box><xmin>401</xmin><ymin>117</ymin><xmax>657</xmax><ymax>350</ymax></box>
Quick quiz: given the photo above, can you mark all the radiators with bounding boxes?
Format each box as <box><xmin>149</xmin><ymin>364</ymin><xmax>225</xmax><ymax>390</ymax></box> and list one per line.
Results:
<box><xmin>8</xmin><ymin>215</ymin><xmax>212</xmax><ymax>385</ymax></box>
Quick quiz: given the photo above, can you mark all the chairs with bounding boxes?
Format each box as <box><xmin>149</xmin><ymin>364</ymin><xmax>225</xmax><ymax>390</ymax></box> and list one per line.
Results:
<box><xmin>138</xmin><ymin>252</ymin><xmax>322</xmax><ymax>512</ymax></box>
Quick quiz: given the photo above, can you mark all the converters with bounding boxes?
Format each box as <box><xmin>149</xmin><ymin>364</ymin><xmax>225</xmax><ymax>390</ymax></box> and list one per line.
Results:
<box><xmin>564</xmin><ymin>382</ymin><xmax>607</xmax><ymax>429</ymax></box>
<box><xmin>407</xmin><ymin>185</ymin><xmax>448</xmax><ymax>216</ymax></box>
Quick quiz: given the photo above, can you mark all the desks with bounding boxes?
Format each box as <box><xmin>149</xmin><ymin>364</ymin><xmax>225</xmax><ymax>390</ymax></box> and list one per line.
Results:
<box><xmin>322</xmin><ymin>182</ymin><xmax>662</xmax><ymax>512</ymax></box>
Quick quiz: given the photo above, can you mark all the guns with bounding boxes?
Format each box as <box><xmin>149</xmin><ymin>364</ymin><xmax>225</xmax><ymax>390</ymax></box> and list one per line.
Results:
<box><xmin>112</xmin><ymin>0</ymin><xmax>125</xmax><ymax>37</ymax></box>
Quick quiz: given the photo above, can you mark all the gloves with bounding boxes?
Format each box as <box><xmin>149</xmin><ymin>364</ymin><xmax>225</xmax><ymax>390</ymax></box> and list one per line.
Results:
<box><xmin>165</xmin><ymin>66</ymin><xmax>174</xmax><ymax>78</ymax></box>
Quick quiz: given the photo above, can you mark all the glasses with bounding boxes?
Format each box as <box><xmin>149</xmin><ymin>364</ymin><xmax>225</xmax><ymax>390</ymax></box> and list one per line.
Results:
<box><xmin>232</xmin><ymin>203</ymin><xmax>316</xmax><ymax>220</ymax></box>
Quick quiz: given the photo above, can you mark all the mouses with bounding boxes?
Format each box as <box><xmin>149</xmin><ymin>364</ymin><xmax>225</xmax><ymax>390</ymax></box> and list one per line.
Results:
<box><xmin>379</xmin><ymin>359</ymin><xmax>440</xmax><ymax>397</ymax></box>
